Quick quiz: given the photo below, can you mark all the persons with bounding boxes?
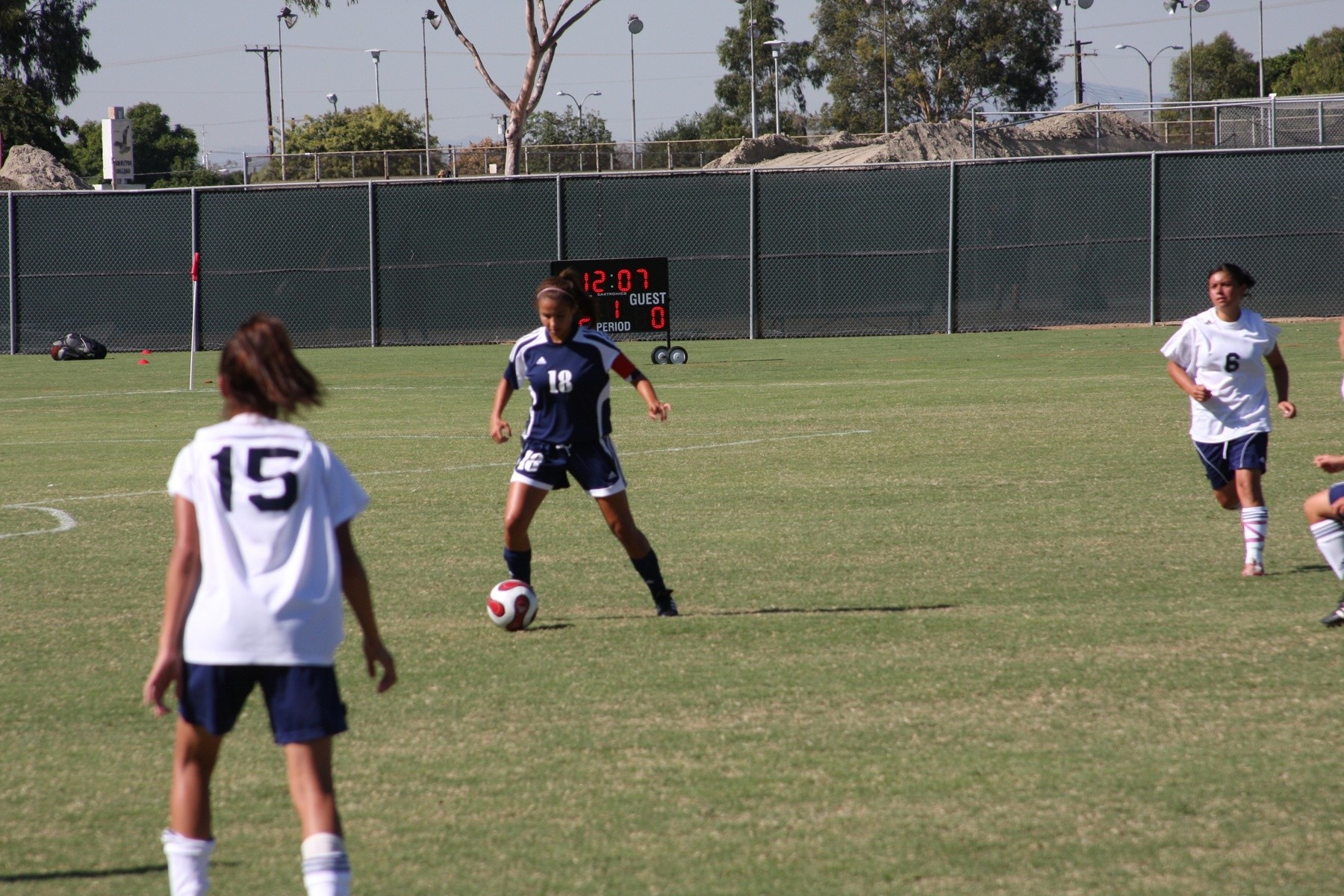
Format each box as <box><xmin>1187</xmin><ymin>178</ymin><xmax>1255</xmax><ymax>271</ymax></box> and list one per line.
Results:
<box><xmin>1160</xmin><ymin>261</ymin><xmax>1297</xmax><ymax>578</ymax></box>
<box><xmin>1302</xmin><ymin>318</ymin><xmax>1344</xmax><ymax>629</ymax></box>
<box><xmin>487</xmin><ymin>267</ymin><xmax>680</xmax><ymax>617</ymax></box>
<box><xmin>140</xmin><ymin>313</ymin><xmax>398</xmax><ymax>896</ymax></box>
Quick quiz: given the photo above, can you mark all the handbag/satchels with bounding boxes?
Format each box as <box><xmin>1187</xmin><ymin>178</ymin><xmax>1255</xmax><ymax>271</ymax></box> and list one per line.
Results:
<box><xmin>52</xmin><ymin>332</ymin><xmax>107</xmax><ymax>361</ymax></box>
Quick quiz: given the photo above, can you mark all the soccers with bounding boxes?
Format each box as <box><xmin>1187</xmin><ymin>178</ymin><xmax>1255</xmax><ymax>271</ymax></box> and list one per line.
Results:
<box><xmin>486</xmin><ymin>579</ymin><xmax>539</xmax><ymax>631</ymax></box>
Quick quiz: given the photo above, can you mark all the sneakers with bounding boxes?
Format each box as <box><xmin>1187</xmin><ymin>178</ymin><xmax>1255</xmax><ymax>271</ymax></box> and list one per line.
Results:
<box><xmin>655</xmin><ymin>589</ymin><xmax>679</xmax><ymax>616</ymax></box>
<box><xmin>1242</xmin><ymin>564</ymin><xmax>1265</xmax><ymax>577</ymax></box>
<box><xmin>1321</xmin><ymin>601</ymin><xmax>1344</xmax><ymax>627</ymax></box>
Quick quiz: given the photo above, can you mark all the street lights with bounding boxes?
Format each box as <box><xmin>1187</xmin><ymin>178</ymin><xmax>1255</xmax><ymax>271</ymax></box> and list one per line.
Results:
<box><xmin>1114</xmin><ymin>43</ymin><xmax>1184</xmax><ymax>138</ymax></box>
<box><xmin>556</xmin><ymin>89</ymin><xmax>602</xmax><ymax>143</ymax></box>
<box><xmin>364</xmin><ymin>49</ymin><xmax>388</xmax><ymax>104</ymax></box>
<box><xmin>762</xmin><ymin>39</ymin><xmax>788</xmax><ymax>135</ymax></box>
